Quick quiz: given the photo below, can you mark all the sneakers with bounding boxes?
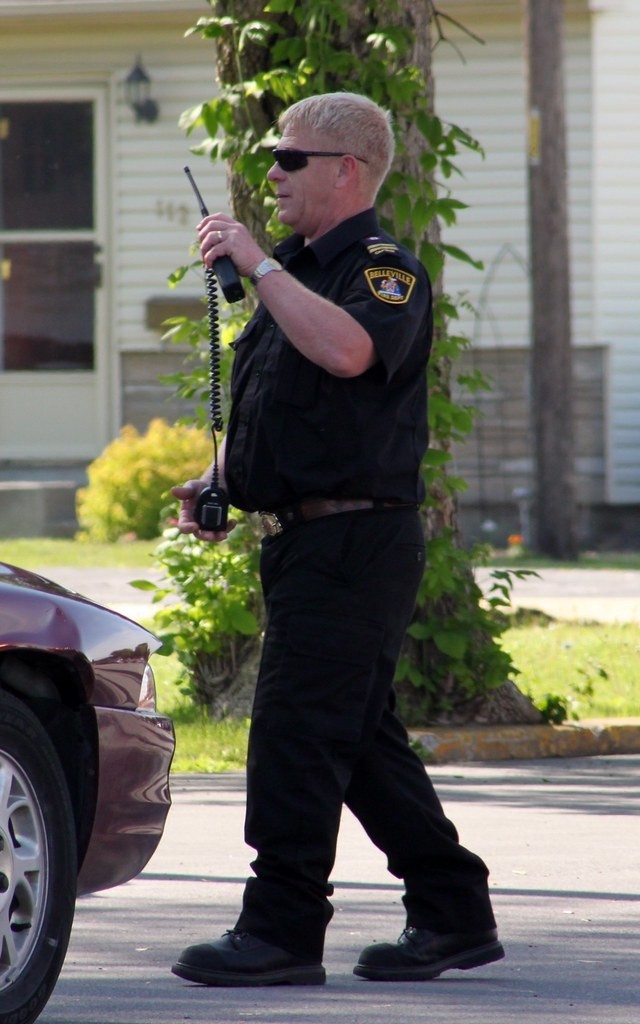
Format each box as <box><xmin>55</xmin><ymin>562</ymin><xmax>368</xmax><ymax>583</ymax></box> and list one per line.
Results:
<box><xmin>172</xmin><ymin>929</ymin><xmax>327</xmax><ymax>987</ymax></box>
<box><xmin>353</xmin><ymin>923</ymin><xmax>505</xmax><ymax>981</ymax></box>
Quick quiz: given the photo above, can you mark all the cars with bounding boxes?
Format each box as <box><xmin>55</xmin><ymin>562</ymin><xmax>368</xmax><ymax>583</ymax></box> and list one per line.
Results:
<box><xmin>0</xmin><ymin>560</ymin><xmax>175</xmax><ymax>1024</ymax></box>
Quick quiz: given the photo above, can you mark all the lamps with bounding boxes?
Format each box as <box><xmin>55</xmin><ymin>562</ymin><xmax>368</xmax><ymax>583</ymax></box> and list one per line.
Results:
<box><xmin>124</xmin><ymin>52</ymin><xmax>159</xmax><ymax>125</ymax></box>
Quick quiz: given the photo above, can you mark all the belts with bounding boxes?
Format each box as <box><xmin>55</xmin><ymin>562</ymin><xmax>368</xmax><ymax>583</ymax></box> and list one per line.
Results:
<box><xmin>259</xmin><ymin>499</ymin><xmax>411</xmax><ymax>536</ymax></box>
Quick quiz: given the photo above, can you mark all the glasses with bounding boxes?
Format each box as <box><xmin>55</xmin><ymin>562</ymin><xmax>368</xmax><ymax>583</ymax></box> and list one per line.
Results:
<box><xmin>272</xmin><ymin>149</ymin><xmax>369</xmax><ymax>171</ymax></box>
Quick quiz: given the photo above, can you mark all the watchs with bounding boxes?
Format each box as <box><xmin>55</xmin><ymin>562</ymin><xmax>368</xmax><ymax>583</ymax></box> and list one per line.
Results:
<box><xmin>249</xmin><ymin>257</ymin><xmax>282</xmax><ymax>286</ymax></box>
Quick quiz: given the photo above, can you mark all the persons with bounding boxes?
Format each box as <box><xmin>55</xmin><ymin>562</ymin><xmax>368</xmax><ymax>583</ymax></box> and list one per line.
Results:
<box><xmin>171</xmin><ymin>93</ymin><xmax>504</xmax><ymax>988</ymax></box>
